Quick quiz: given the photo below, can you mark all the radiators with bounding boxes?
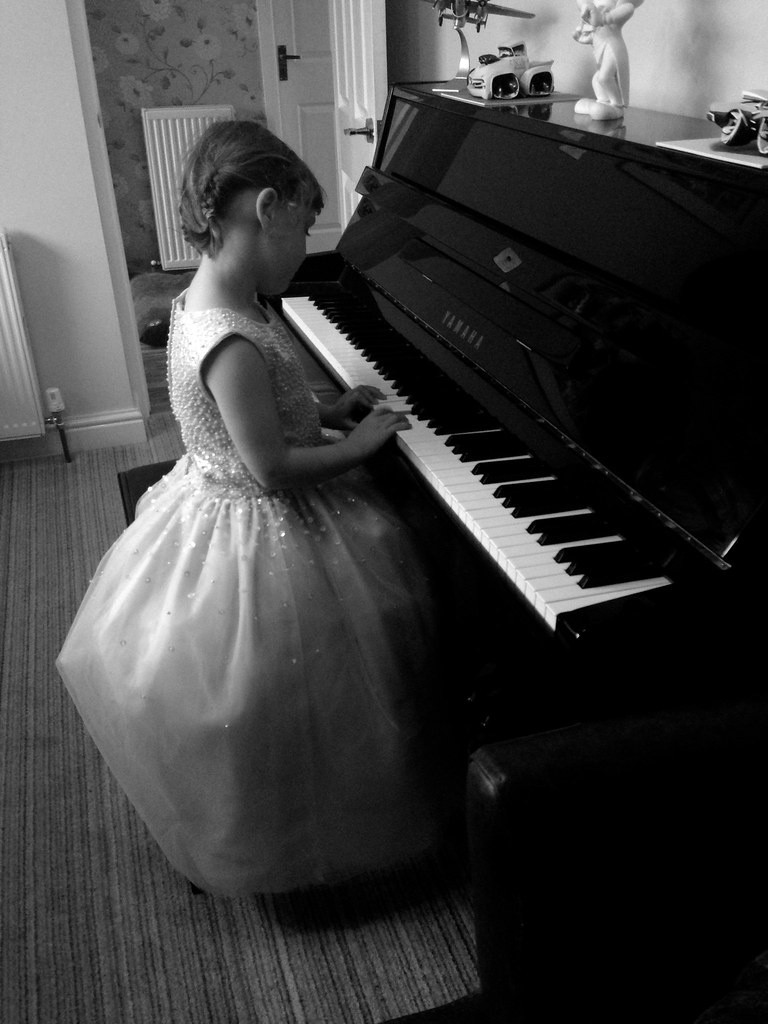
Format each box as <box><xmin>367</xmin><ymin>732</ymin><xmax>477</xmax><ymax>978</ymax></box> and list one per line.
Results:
<box><xmin>0</xmin><ymin>232</ymin><xmax>74</xmax><ymax>465</ymax></box>
<box><xmin>141</xmin><ymin>103</ymin><xmax>236</xmax><ymax>274</ymax></box>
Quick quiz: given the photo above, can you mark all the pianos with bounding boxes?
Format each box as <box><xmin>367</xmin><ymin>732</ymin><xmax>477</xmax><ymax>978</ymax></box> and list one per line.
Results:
<box><xmin>270</xmin><ymin>78</ymin><xmax>768</xmax><ymax>882</ymax></box>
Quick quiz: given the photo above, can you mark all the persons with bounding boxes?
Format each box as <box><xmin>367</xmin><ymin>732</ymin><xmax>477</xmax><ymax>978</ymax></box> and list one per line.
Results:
<box><xmin>164</xmin><ymin>119</ymin><xmax>418</xmax><ymax>898</ymax></box>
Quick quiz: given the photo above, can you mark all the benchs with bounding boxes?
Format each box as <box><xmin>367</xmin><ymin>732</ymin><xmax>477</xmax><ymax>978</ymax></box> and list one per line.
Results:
<box><xmin>117</xmin><ymin>459</ymin><xmax>177</xmax><ymax>525</ymax></box>
